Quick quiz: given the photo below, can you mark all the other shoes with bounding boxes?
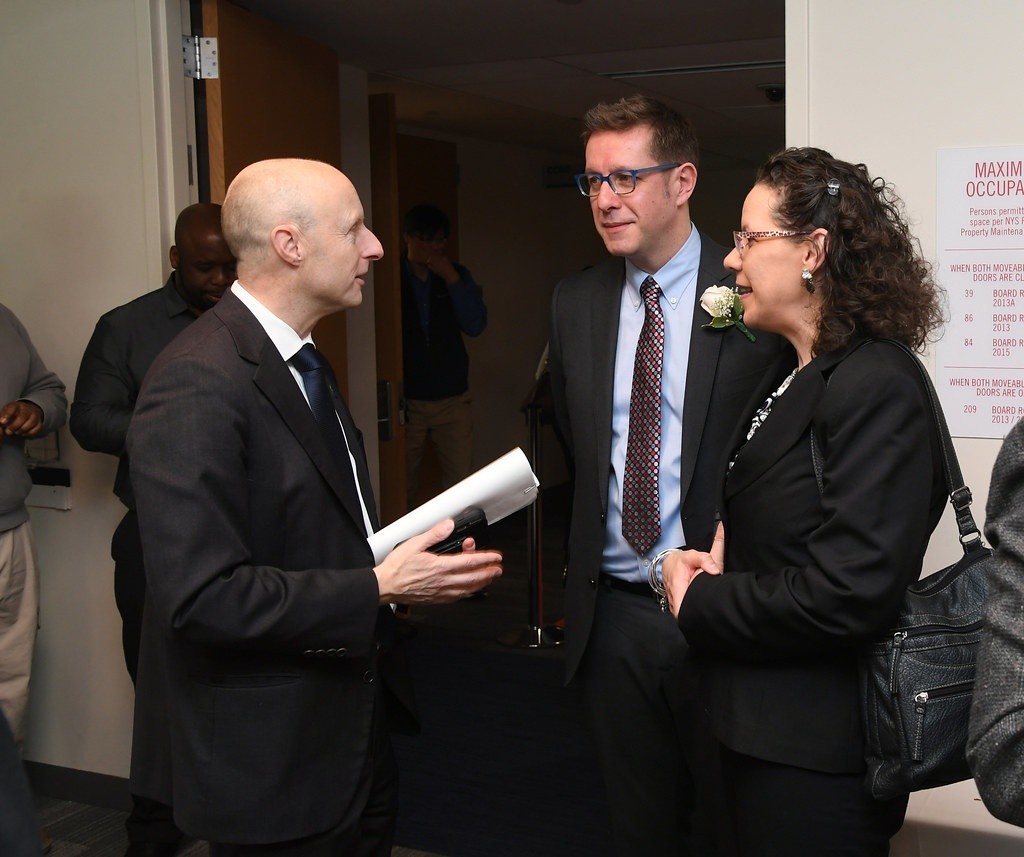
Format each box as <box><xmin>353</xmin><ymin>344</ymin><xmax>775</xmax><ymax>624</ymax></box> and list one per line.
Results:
<box><xmin>128</xmin><ymin>807</ymin><xmax>200</xmax><ymax>856</ymax></box>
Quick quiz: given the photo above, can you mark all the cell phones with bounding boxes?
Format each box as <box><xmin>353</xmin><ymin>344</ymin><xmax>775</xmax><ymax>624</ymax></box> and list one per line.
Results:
<box><xmin>391</xmin><ymin>506</ymin><xmax>491</xmax><ymax>555</ymax></box>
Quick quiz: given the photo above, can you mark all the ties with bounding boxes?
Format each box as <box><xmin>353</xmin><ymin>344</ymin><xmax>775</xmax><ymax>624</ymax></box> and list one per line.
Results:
<box><xmin>293</xmin><ymin>343</ymin><xmax>368</xmax><ymax>543</ymax></box>
<box><xmin>621</xmin><ymin>274</ymin><xmax>665</xmax><ymax>557</ymax></box>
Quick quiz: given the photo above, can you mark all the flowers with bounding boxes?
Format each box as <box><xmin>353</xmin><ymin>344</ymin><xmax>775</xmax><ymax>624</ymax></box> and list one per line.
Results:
<box><xmin>699</xmin><ymin>285</ymin><xmax>758</xmax><ymax>342</ymax></box>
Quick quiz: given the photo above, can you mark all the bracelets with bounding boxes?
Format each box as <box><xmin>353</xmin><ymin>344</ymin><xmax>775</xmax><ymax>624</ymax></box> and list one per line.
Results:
<box><xmin>646</xmin><ymin>546</ymin><xmax>690</xmax><ymax>614</ymax></box>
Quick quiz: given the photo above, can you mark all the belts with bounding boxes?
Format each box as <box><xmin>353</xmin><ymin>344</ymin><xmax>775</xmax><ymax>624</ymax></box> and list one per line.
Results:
<box><xmin>502</xmin><ymin>624</ymin><xmax>568</xmax><ymax>650</ymax></box>
<box><xmin>604</xmin><ymin>575</ymin><xmax>669</xmax><ymax>600</ymax></box>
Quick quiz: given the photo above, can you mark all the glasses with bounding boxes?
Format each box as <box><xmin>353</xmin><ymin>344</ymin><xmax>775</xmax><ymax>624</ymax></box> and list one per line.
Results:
<box><xmin>733</xmin><ymin>230</ymin><xmax>814</xmax><ymax>256</ymax></box>
<box><xmin>414</xmin><ymin>235</ymin><xmax>446</xmax><ymax>244</ymax></box>
<box><xmin>574</xmin><ymin>162</ymin><xmax>682</xmax><ymax>197</ymax></box>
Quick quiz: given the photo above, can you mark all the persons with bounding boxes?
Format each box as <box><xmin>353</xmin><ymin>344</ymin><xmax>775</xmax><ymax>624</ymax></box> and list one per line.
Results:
<box><xmin>518</xmin><ymin>93</ymin><xmax>801</xmax><ymax>857</ymax></box>
<box><xmin>0</xmin><ymin>295</ymin><xmax>68</xmax><ymax>855</ymax></box>
<box><xmin>645</xmin><ymin>145</ymin><xmax>949</xmax><ymax>857</ymax></box>
<box><xmin>123</xmin><ymin>155</ymin><xmax>503</xmax><ymax>855</ymax></box>
<box><xmin>392</xmin><ymin>206</ymin><xmax>494</xmax><ymax>597</ymax></box>
<box><xmin>67</xmin><ymin>199</ymin><xmax>274</xmax><ymax>696</ymax></box>
<box><xmin>961</xmin><ymin>413</ymin><xmax>1024</xmax><ymax>827</ymax></box>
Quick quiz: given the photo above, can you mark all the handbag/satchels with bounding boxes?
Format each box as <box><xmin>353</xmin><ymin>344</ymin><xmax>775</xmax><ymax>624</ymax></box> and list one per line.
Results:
<box><xmin>852</xmin><ymin>542</ymin><xmax>995</xmax><ymax>804</ymax></box>
